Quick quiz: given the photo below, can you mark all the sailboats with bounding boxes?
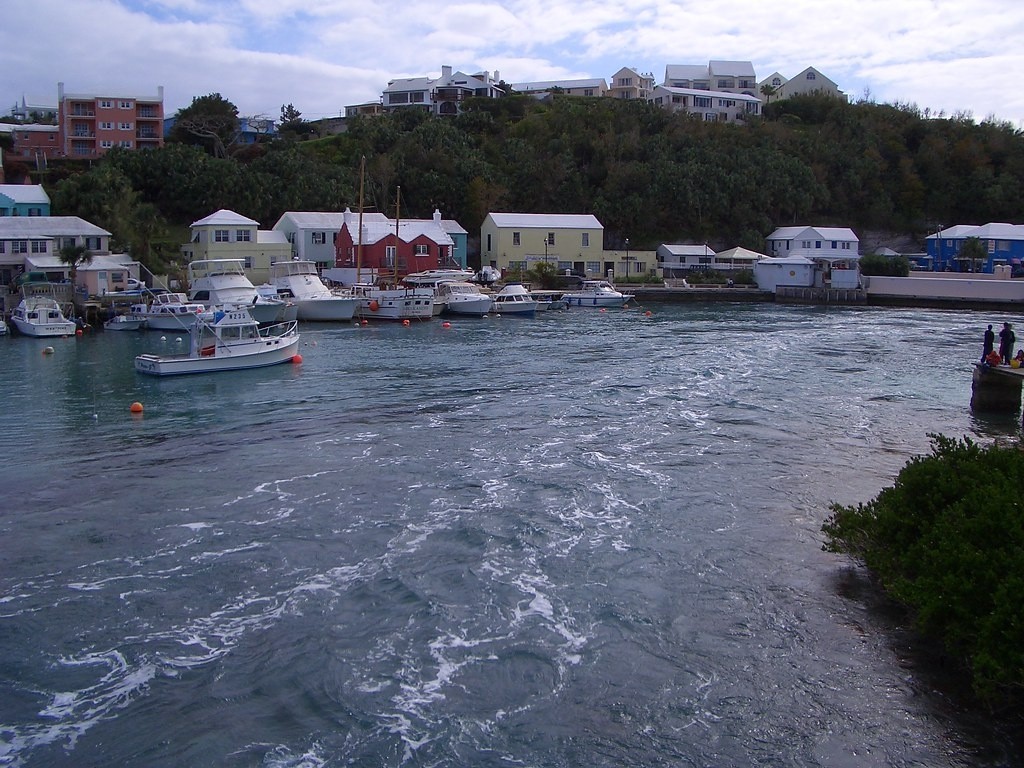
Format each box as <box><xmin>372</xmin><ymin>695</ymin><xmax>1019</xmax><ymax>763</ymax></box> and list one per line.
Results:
<box><xmin>330</xmin><ymin>154</ymin><xmax>434</xmax><ymax>319</ymax></box>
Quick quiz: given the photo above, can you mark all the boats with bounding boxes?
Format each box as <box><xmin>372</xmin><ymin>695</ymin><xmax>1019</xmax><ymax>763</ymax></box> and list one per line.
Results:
<box><xmin>11</xmin><ymin>282</ymin><xmax>76</xmax><ymax>336</ymax></box>
<box><xmin>253</xmin><ymin>257</ymin><xmax>362</xmax><ymax>322</ymax></box>
<box><xmin>274</xmin><ymin>302</ymin><xmax>299</xmax><ymax>322</ymax></box>
<box><xmin>134</xmin><ymin>304</ymin><xmax>301</xmax><ymax>376</ymax></box>
<box><xmin>402</xmin><ymin>267</ymin><xmax>474</xmax><ymax>284</ymax></box>
<box><xmin>434</xmin><ymin>279</ymin><xmax>492</xmax><ymax>315</ymax></box>
<box><xmin>103</xmin><ymin>315</ymin><xmax>147</xmax><ymax>331</ymax></box>
<box><xmin>560</xmin><ymin>281</ymin><xmax>635</xmax><ymax>307</ymax></box>
<box><xmin>488</xmin><ymin>285</ymin><xmax>552</xmax><ymax>312</ymax></box>
<box><xmin>130</xmin><ymin>293</ymin><xmax>206</xmax><ymax>329</ymax></box>
<box><xmin>187</xmin><ymin>259</ymin><xmax>282</xmax><ymax>329</ymax></box>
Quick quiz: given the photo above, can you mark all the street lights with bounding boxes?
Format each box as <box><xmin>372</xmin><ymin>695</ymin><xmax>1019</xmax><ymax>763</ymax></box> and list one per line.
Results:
<box><xmin>625</xmin><ymin>238</ymin><xmax>629</xmax><ymax>278</ymax></box>
<box><xmin>704</xmin><ymin>242</ymin><xmax>708</xmax><ymax>282</ymax></box>
<box><xmin>543</xmin><ymin>238</ymin><xmax>547</xmax><ymax>285</ymax></box>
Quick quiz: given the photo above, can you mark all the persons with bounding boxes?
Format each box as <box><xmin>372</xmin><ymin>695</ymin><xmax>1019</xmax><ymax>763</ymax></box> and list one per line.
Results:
<box><xmin>980</xmin><ymin>324</ymin><xmax>994</xmax><ymax>367</ymax></box>
<box><xmin>1015</xmin><ymin>349</ymin><xmax>1024</xmax><ymax>368</ymax></box>
<box><xmin>999</xmin><ymin>322</ymin><xmax>1016</xmax><ymax>365</ymax></box>
